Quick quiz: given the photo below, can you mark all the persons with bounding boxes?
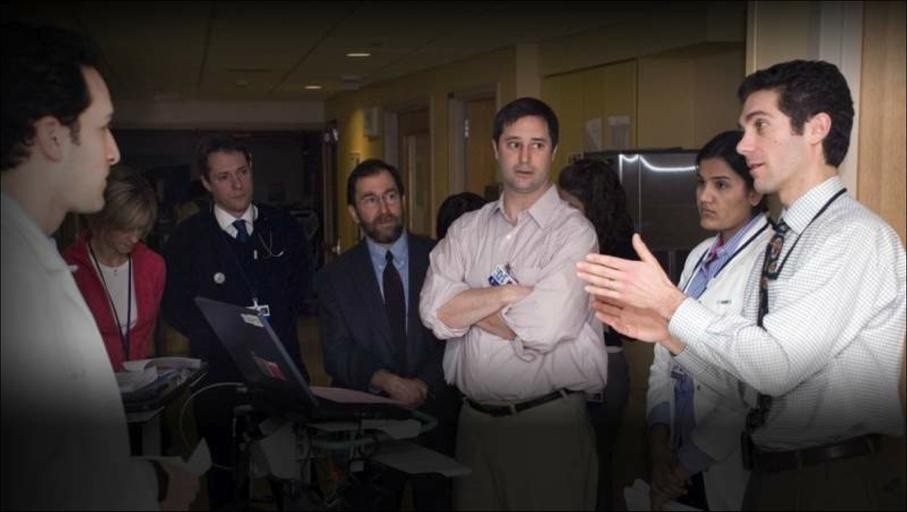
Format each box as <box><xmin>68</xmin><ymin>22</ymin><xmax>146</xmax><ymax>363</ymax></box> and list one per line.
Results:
<box><xmin>416</xmin><ymin>94</ymin><xmax>612</xmax><ymax>512</ymax></box>
<box><xmin>158</xmin><ymin>141</ymin><xmax>313</xmax><ymax>510</ymax></box>
<box><xmin>435</xmin><ymin>190</ymin><xmax>484</xmax><ymax>247</ymax></box>
<box><xmin>312</xmin><ymin>159</ymin><xmax>450</xmax><ymax>511</ymax></box>
<box><xmin>574</xmin><ymin>59</ymin><xmax>905</xmax><ymax>512</ymax></box>
<box><xmin>61</xmin><ymin>165</ymin><xmax>170</xmax><ymax>456</ymax></box>
<box><xmin>556</xmin><ymin>159</ymin><xmax>628</xmax><ymax>510</ymax></box>
<box><xmin>0</xmin><ymin>27</ymin><xmax>199</xmax><ymax>508</ymax></box>
<box><xmin>632</xmin><ymin>128</ymin><xmax>777</xmax><ymax>509</ymax></box>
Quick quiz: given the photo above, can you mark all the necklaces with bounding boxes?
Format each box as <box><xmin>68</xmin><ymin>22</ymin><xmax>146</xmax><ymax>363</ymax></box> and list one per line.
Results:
<box><xmin>96</xmin><ymin>251</ymin><xmax>126</xmax><ymax>276</ymax></box>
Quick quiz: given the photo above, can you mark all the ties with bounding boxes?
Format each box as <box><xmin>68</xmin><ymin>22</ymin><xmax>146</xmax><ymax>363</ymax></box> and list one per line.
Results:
<box><xmin>233</xmin><ymin>219</ymin><xmax>250</xmax><ymax>242</ymax></box>
<box><xmin>745</xmin><ymin>221</ymin><xmax>790</xmax><ymax>433</ymax></box>
<box><xmin>383</xmin><ymin>249</ymin><xmax>409</xmax><ymax>378</ymax></box>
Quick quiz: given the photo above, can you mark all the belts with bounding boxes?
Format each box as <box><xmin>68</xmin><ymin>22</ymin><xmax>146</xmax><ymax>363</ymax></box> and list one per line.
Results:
<box><xmin>464</xmin><ymin>388</ymin><xmax>575</xmax><ymax>418</ymax></box>
<box><xmin>756</xmin><ymin>432</ymin><xmax>889</xmax><ymax>474</ymax></box>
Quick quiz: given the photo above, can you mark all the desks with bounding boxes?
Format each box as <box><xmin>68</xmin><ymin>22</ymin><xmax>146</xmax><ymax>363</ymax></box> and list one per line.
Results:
<box><xmin>123</xmin><ymin>358</ymin><xmax>208</xmax><ymax>457</ymax></box>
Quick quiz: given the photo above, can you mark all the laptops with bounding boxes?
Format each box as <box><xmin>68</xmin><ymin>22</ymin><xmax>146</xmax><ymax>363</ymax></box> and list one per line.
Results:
<box><xmin>193</xmin><ymin>296</ymin><xmax>415</xmax><ymax>419</ymax></box>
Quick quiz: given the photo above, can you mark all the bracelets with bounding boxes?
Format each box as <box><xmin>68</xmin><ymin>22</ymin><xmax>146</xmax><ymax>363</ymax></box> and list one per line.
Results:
<box><xmin>145</xmin><ymin>457</ymin><xmax>171</xmax><ymax>501</ymax></box>
